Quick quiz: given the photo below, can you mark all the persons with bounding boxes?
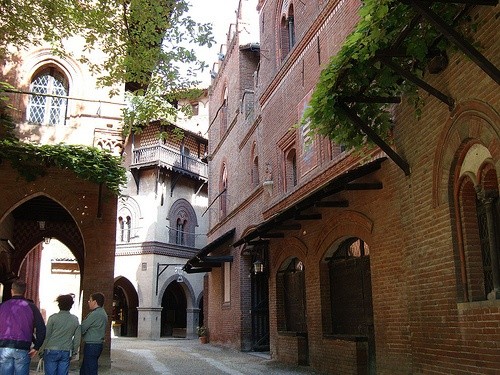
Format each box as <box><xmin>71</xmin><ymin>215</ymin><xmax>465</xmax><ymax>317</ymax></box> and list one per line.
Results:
<box><xmin>38</xmin><ymin>295</ymin><xmax>81</xmax><ymax>375</ymax></box>
<box><xmin>79</xmin><ymin>292</ymin><xmax>108</xmax><ymax>375</ymax></box>
<box><xmin>0</xmin><ymin>279</ymin><xmax>46</xmax><ymax>375</ymax></box>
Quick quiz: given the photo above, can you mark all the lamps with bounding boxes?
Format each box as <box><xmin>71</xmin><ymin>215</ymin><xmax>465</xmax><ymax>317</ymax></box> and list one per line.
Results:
<box><xmin>253</xmin><ymin>260</ymin><xmax>264</xmax><ymax>274</ymax></box>
<box><xmin>174</xmin><ymin>266</ymin><xmax>185</xmax><ymax>283</ymax></box>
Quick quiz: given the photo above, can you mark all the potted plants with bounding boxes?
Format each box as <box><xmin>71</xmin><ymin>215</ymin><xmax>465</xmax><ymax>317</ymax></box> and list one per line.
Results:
<box><xmin>194</xmin><ymin>325</ymin><xmax>209</xmax><ymax>344</ymax></box>
